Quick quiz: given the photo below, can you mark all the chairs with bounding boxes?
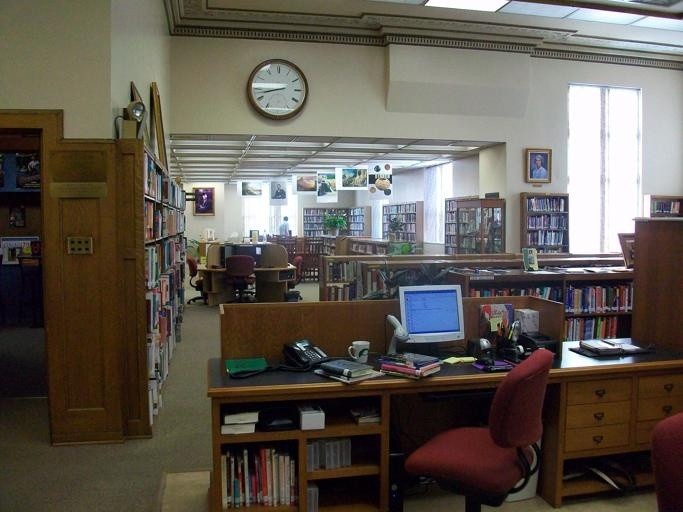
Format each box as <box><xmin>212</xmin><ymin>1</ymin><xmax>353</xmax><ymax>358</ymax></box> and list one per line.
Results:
<box><xmin>185</xmin><ymin>255</ymin><xmax>208</xmax><ymax>306</ymax></box>
<box><xmin>221</xmin><ymin>254</ymin><xmax>259</xmax><ymax>303</ymax></box>
<box><xmin>404</xmin><ymin>348</ymin><xmax>556</xmax><ymax>510</ymax></box>
<box><xmin>648</xmin><ymin>411</ymin><xmax>680</xmax><ymax>512</ymax></box>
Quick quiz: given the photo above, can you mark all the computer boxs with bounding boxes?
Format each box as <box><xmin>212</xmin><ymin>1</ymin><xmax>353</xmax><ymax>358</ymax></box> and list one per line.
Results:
<box><xmin>481</xmin><ymin>421</ymin><xmax>541</xmax><ymax>502</ymax></box>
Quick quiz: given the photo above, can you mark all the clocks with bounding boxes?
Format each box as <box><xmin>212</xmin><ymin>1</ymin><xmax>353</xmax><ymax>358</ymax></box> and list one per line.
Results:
<box><xmin>245</xmin><ymin>58</ymin><xmax>308</xmax><ymax>122</ymax></box>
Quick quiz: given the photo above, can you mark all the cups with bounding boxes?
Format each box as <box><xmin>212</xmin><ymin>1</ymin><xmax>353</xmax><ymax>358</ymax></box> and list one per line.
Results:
<box><xmin>199</xmin><ymin>256</ymin><xmax>206</xmax><ymax>265</ymax></box>
<box><xmin>348</xmin><ymin>341</ymin><xmax>369</xmax><ymax>364</ymax></box>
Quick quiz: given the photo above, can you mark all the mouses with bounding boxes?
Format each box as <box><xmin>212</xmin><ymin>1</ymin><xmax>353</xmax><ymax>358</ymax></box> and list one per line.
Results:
<box><xmin>475</xmin><ymin>357</ymin><xmax>494</xmax><ymax>366</ymax></box>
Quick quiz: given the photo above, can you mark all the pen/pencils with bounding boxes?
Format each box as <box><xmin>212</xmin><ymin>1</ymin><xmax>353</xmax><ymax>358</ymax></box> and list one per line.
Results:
<box><xmin>497</xmin><ymin>318</ymin><xmax>515</xmax><ymax>340</ymax></box>
<box><xmin>601</xmin><ymin>339</ymin><xmax>615</xmax><ymax>346</ymax></box>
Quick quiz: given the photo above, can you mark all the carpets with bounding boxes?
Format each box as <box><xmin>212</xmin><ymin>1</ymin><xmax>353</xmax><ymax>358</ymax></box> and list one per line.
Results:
<box><xmin>152</xmin><ymin>467</ymin><xmax>661</xmax><ymax>511</ymax></box>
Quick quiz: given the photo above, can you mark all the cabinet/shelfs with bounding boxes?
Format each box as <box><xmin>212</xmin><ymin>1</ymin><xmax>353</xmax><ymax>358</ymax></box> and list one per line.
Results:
<box><xmin>112</xmin><ymin>135</ymin><xmax>188</xmax><ymax>440</ymax></box>
<box><xmin>197</xmin><ymin>338</ymin><xmax>682</xmax><ymax>511</ymax></box>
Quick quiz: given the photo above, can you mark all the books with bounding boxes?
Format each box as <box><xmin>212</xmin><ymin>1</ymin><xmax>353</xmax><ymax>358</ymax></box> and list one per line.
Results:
<box><xmin>347</xmin><ymin>404</ymin><xmax>381</xmax><ymax>424</ymax></box>
<box><xmin>569</xmin><ymin>339</ymin><xmax>650</xmax><ymax>357</ymax></box>
<box><xmin>469</xmin><ymin>282</ymin><xmax>564</xmax><ymax>303</ymax></box>
<box><xmin>314</xmin><ymin>369</ymin><xmax>384</xmax><ymax>385</ymax></box>
<box><xmin>321</xmin><ymin>368</ymin><xmax>369</xmax><ymax>381</ymax></box>
<box><xmin>381</xmin><ymin>363</ymin><xmax>441</xmax><ymax>377</ymax></box>
<box><xmin>379</xmin><ymin>369</ymin><xmax>420</xmax><ymax>380</ymax></box>
<box><xmin>378</xmin><ymin>358</ymin><xmax>439</xmax><ymax>369</ymax></box>
<box><xmin>141</xmin><ymin>153</ymin><xmax>187</xmax><ymax>426</ymax></box>
<box><xmin>564</xmin><ymin>277</ymin><xmax>632</xmax><ymax>341</ymax></box>
<box><xmin>320</xmin><ymin>359</ymin><xmax>373</xmax><ymax>377</ymax></box>
<box><xmin>304</xmin><ymin>195</ymin><xmax>570</xmax><ymax>254</ymax></box>
<box><xmin>218</xmin><ymin>400</ymin><xmax>300</xmax><ymax>512</ymax></box>
<box><xmin>383</xmin><ymin>351</ymin><xmax>438</xmax><ymax>365</ymax></box>
<box><xmin>651</xmin><ymin>199</ymin><xmax>682</xmax><ymax>216</ymax></box>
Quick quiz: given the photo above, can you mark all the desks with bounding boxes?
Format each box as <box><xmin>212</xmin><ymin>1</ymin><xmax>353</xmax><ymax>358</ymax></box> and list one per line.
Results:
<box><xmin>192</xmin><ymin>242</ymin><xmax>295</xmax><ymax>310</ymax></box>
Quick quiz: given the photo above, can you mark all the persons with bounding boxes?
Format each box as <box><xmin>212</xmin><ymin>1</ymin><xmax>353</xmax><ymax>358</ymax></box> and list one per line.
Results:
<box><xmin>271</xmin><ymin>183</ymin><xmax>285</xmax><ymax>199</ymax></box>
<box><xmin>528</xmin><ymin>152</ymin><xmax>548</xmax><ymax>180</ymax></box>
<box><xmin>193</xmin><ymin>189</ymin><xmax>213</xmax><ymax>214</ymax></box>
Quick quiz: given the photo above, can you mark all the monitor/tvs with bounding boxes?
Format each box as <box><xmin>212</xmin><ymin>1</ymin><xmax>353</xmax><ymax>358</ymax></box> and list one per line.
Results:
<box><xmin>399</xmin><ymin>285</ymin><xmax>464</xmax><ymax>356</ymax></box>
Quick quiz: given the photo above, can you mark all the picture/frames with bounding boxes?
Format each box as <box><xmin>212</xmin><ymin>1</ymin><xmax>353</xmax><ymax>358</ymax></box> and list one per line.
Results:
<box><xmin>523</xmin><ymin>146</ymin><xmax>551</xmax><ymax>184</ymax></box>
<box><xmin>191</xmin><ymin>186</ymin><xmax>215</xmax><ymax>217</ymax></box>
<box><xmin>130</xmin><ymin>79</ymin><xmax>168</xmax><ymax>171</ymax></box>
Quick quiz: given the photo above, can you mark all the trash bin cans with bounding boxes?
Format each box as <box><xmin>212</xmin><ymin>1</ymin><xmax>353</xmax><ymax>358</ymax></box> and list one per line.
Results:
<box><xmin>284</xmin><ymin>291</ymin><xmax>300</xmax><ymax>302</ymax></box>
<box><xmin>505</xmin><ymin>437</ymin><xmax>541</xmax><ymax>502</ymax></box>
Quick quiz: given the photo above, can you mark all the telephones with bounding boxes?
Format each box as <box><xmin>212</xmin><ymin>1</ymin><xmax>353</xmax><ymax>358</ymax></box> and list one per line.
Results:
<box><xmin>282</xmin><ymin>338</ymin><xmax>330</xmax><ymax>369</ymax></box>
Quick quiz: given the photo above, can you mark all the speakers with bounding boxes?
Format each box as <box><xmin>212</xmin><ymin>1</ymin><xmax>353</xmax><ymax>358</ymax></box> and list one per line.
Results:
<box><xmin>468</xmin><ymin>337</ymin><xmax>493</xmax><ymax>359</ymax></box>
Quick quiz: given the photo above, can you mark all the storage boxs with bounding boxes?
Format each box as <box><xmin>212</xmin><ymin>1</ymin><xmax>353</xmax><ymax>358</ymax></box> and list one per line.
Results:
<box><xmin>297</xmin><ymin>404</ymin><xmax>325</xmax><ymax>432</ymax></box>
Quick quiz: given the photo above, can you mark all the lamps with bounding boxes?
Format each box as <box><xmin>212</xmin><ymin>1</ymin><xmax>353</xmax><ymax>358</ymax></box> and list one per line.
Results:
<box><xmin>114</xmin><ymin>98</ymin><xmax>145</xmax><ymax>138</ymax></box>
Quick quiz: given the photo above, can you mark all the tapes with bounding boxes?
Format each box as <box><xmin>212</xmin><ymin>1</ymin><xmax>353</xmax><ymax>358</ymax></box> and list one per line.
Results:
<box><xmin>516</xmin><ymin>345</ymin><xmax>524</xmax><ymax>354</ymax></box>
